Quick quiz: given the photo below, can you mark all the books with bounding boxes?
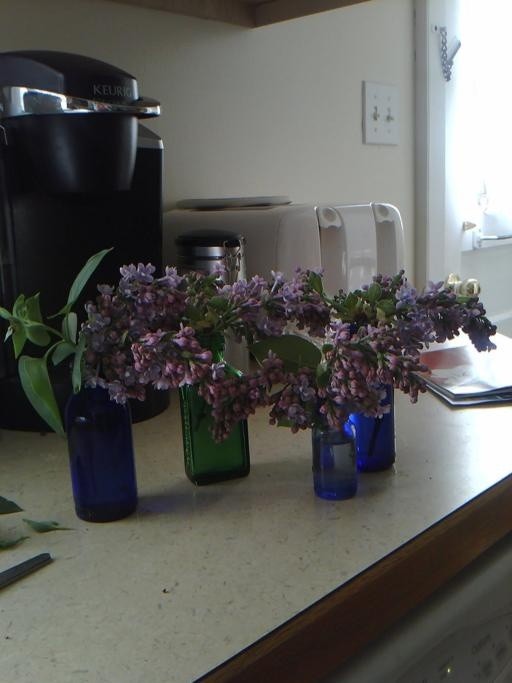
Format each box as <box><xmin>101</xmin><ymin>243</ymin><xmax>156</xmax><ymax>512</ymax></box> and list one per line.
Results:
<box><xmin>388</xmin><ymin>325</ymin><xmax>512</xmax><ymax>409</ymax></box>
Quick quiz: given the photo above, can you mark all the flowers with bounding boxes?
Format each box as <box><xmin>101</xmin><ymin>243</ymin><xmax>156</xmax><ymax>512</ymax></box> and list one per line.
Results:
<box><xmin>0</xmin><ymin>240</ymin><xmax>496</xmax><ymax>436</ymax></box>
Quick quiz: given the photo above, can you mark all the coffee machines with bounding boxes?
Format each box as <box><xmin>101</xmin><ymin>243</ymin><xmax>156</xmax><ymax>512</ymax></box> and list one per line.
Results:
<box><xmin>0</xmin><ymin>40</ymin><xmax>175</xmax><ymax>434</ymax></box>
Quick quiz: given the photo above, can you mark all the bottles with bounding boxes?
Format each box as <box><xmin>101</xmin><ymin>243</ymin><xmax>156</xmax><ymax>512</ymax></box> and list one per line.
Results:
<box><xmin>342</xmin><ymin>319</ymin><xmax>396</xmax><ymax>473</ymax></box>
<box><xmin>174</xmin><ymin>227</ymin><xmax>250</xmax><ymax>298</ymax></box>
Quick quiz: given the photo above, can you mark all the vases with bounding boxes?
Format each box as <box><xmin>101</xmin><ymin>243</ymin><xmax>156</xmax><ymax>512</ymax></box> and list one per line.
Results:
<box><xmin>345</xmin><ymin>381</ymin><xmax>394</xmax><ymax>472</ymax></box>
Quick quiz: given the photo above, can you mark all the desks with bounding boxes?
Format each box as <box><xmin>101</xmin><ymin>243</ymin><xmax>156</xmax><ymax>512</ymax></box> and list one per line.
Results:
<box><xmin>0</xmin><ymin>326</ymin><xmax>511</xmax><ymax>680</ymax></box>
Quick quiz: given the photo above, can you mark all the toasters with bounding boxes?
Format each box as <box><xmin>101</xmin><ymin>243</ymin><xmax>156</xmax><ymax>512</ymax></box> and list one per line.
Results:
<box><xmin>164</xmin><ymin>188</ymin><xmax>410</xmax><ymax>308</ymax></box>
